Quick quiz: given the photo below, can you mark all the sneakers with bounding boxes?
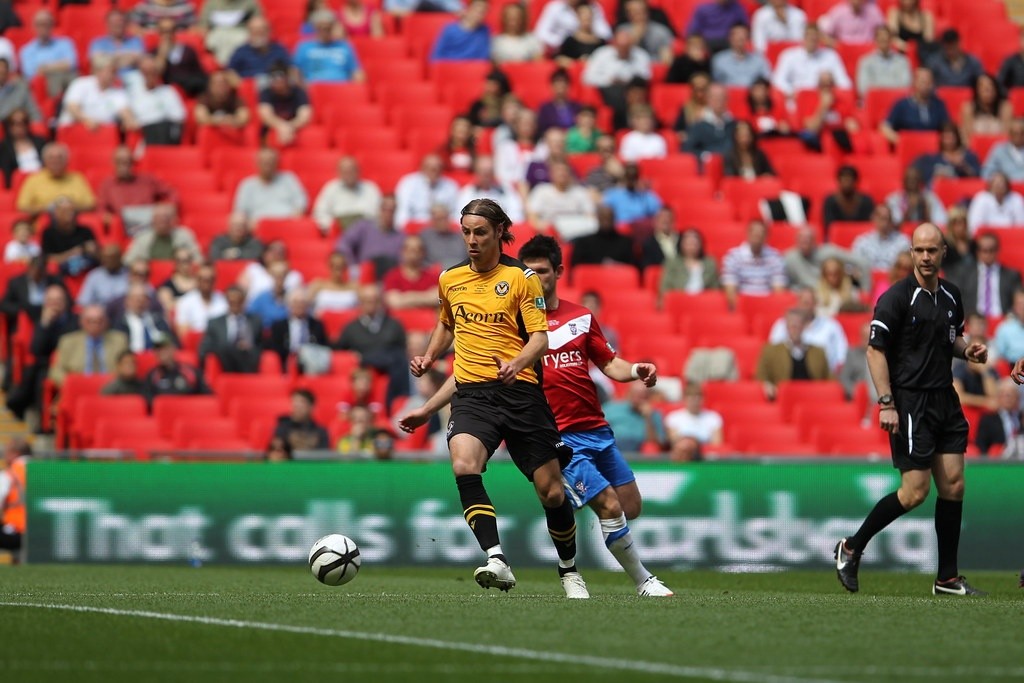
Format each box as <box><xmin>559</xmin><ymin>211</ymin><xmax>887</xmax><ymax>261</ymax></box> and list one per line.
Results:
<box><xmin>834</xmin><ymin>536</ymin><xmax>860</xmax><ymax>592</ymax></box>
<box><xmin>560</xmin><ymin>572</ymin><xmax>590</xmax><ymax>599</ymax></box>
<box><xmin>933</xmin><ymin>575</ymin><xmax>989</xmax><ymax>599</ymax></box>
<box><xmin>636</xmin><ymin>576</ymin><xmax>675</xmax><ymax>597</ymax></box>
<box><xmin>474</xmin><ymin>557</ymin><xmax>516</xmax><ymax>593</ymax></box>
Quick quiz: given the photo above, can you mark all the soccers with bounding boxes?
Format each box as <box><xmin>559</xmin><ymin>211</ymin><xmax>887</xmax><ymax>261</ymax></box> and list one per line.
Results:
<box><xmin>307</xmin><ymin>533</ymin><xmax>363</xmax><ymax>587</ymax></box>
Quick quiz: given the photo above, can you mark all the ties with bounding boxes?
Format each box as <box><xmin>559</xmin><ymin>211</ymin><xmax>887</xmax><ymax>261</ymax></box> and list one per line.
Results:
<box><xmin>94</xmin><ymin>338</ymin><xmax>99</xmax><ymax>374</ymax></box>
<box><xmin>236</xmin><ymin>314</ymin><xmax>242</xmax><ymax>342</ymax></box>
<box><xmin>140</xmin><ymin>314</ymin><xmax>152</xmax><ymax>351</ymax></box>
<box><xmin>985</xmin><ymin>266</ymin><xmax>991</xmax><ymax>317</ymax></box>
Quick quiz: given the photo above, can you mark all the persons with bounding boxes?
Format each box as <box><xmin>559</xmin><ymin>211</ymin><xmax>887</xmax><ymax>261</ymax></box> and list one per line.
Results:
<box><xmin>265</xmin><ymin>388</ymin><xmax>329</xmax><ymax>461</ymax></box>
<box><xmin>399</xmin><ymin>235</ymin><xmax>675</xmax><ymax>596</ymax></box>
<box><xmin>0</xmin><ymin>441</ymin><xmax>33</xmax><ymax>562</ymax></box>
<box><xmin>834</xmin><ymin>221</ymin><xmax>989</xmax><ymax>596</ymax></box>
<box><xmin>0</xmin><ymin>0</ymin><xmax>1024</xmax><ymax>460</ymax></box>
<box><xmin>409</xmin><ymin>198</ymin><xmax>590</xmax><ymax>598</ymax></box>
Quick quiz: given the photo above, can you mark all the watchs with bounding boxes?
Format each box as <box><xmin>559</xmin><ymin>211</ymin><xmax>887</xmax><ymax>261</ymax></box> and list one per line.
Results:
<box><xmin>878</xmin><ymin>394</ymin><xmax>894</xmax><ymax>405</ymax></box>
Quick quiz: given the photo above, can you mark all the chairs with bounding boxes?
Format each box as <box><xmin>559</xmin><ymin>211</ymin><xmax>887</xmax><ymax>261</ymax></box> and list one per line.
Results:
<box><xmin>0</xmin><ymin>0</ymin><xmax>1024</xmax><ymax>461</ymax></box>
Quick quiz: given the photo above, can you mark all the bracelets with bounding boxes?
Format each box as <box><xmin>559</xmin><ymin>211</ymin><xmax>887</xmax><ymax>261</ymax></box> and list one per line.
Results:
<box><xmin>879</xmin><ymin>407</ymin><xmax>896</xmax><ymax>412</ymax></box>
<box><xmin>631</xmin><ymin>363</ymin><xmax>641</xmax><ymax>379</ymax></box>
<box><xmin>963</xmin><ymin>347</ymin><xmax>969</xmax><ymax>360</ymax></box>
